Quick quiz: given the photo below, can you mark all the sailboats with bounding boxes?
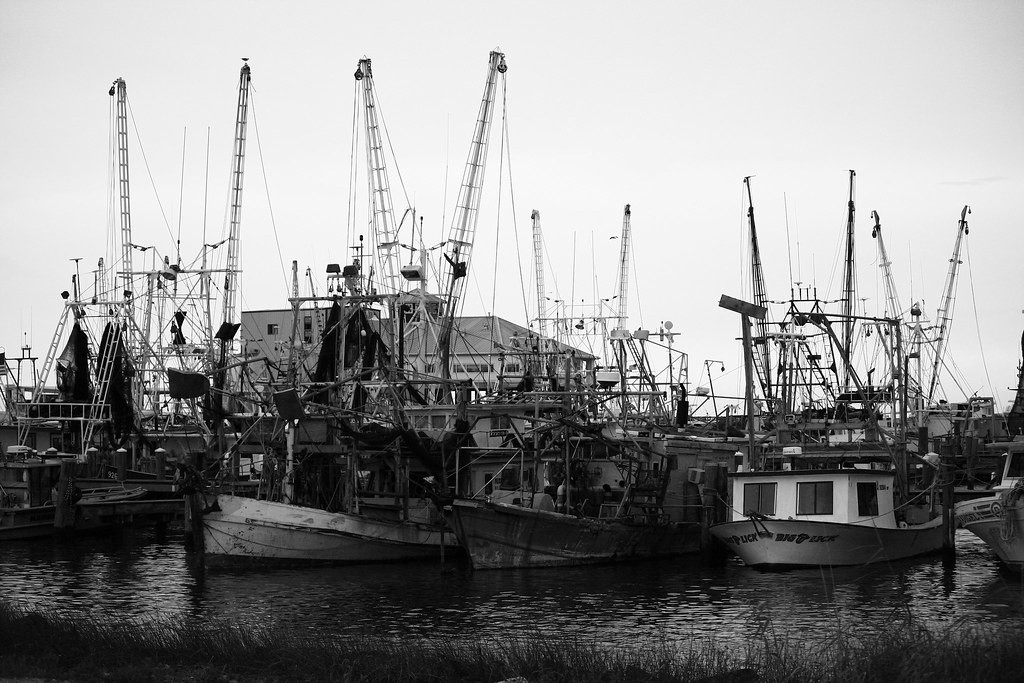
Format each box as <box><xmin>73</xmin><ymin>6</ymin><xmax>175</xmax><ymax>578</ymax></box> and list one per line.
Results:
<box><xmin>0</xmin><ymin>44</ymin><xmax>1024</xmax><ymax>577</ymax></box>
